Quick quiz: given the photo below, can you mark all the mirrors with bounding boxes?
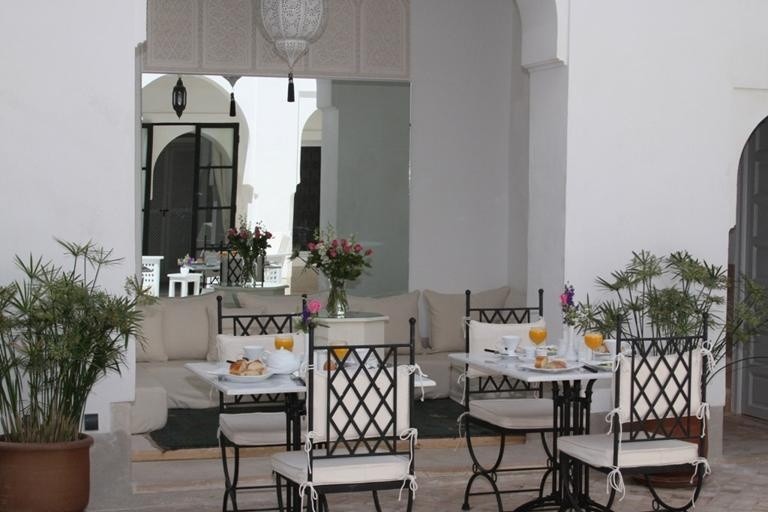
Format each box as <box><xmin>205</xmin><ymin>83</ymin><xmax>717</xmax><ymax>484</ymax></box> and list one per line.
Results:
<box><xmin>138</xmin><ymin>72</ymin><xmax>410</xmax><ymax>309</ymax></box>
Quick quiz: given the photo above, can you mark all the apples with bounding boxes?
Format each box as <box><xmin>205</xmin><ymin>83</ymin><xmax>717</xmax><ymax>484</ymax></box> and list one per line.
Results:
<box><xmin>324</xmin><ymin>359</ymin><xmax>337</xmax><ymax>370</ymax></box>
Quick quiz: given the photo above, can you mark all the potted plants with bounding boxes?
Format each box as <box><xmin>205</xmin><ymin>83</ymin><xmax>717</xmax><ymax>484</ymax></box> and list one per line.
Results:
<box><xmin>0</xmin><ymin>237</ymin><xmax>161</xmax><ymax>512</ymax></box>
<box><xmin>577</xmin><ymin>248</ymin><xmax>768</xmax><ymax>490</ymax></box>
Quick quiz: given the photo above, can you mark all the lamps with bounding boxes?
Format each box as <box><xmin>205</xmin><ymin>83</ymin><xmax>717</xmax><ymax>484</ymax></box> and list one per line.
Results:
<box><xmin>255</xmin><ymin>0</ymin><xmax>328</xmax><ymax>102</ymax></box>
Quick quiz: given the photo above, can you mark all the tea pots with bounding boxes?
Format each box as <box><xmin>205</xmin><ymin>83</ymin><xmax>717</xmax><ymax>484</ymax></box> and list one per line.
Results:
<box><xmin>259</xmin><ymin>346</ymin><xmax>302</xmax><ymax>375</ymax></box>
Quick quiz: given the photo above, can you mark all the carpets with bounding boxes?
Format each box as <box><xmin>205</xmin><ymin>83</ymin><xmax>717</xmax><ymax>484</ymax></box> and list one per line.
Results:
<box><xmin>148</xmin><ymin>396</ymin><xmax>525</xmax><ymax>451</ymax></box>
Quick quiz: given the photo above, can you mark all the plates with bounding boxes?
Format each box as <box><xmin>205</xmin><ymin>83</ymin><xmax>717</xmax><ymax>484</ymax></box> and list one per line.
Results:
<box><xmin>483</xmin><ymin>323</ymin><xmax>629</xmax><ymax>377</ymax></box>
<box><xmin>205</xmin><ymin>364</ymin><xmax>278</xmax><ymax>383</ymax></box>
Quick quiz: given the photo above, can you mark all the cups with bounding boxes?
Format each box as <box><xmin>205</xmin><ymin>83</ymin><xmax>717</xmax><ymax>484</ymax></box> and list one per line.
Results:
<box><xmin>274</xmin><ymin>334</ymin><xmax>295</xmax><ymax>352</ymax></box>
<box><xmin>330</xmin><ymin>340</ymin><xmax>348</xmax><ymax>363</ymax></box>
<box><xmin>243</xmin><ymin>346</ymin><xmax>265</xmax><ymax>363</ymax></box>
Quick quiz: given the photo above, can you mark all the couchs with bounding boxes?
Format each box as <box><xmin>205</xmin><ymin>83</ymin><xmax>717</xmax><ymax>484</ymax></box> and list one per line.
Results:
<box><xmin>131</xmin><ymin>286</ymin><xmax>529</xmax><ymax>452</ymax></box>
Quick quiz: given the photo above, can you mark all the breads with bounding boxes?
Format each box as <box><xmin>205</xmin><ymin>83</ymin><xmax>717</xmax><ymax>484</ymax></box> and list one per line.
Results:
<box><xmin>229</xmin><ymin>359</ymin><xmax>270</xmax><ymax>376</ymax></box>
<box><xmin>535</xmin><ymin>356</ymin><xmax>566</xmax><ymax>369</ymax></box>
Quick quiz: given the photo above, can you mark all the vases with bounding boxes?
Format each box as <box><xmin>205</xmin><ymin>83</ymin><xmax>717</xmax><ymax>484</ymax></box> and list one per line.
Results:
<box><xmin>239</xmin><ymin>258</ymin><xmax>257</xmax><ymax>288</ymax></box>
<box><xmin>326</xmin><ymin>279</ymin><xmax>348</xmax><ymax>318</ymax></box>
<box><xmin>180</xmin><ymin>267</ymin><xmax>189</xmax><ymax>273</ymax></box>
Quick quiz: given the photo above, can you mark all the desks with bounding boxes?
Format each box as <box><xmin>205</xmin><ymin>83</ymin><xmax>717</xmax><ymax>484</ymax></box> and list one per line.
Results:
<box><xmin>167</xmin><ymin>273</ymin><xmax>202</xmax><ymax>297</ymax></box>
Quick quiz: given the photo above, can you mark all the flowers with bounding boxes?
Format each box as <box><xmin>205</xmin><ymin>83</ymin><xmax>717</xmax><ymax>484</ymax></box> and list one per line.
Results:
<box><xmin>227</xmin><ymin>214</ymin><xmax>273</xmax><ymax>259</ymax></box>
<box><xmin>177</xmin><ymin>253</ymin><xmax>194</xmax><ymax>267</ymax></box>
<box><xmin>303</xmin><ymin>220</ymin><xmax>376</xmax><ymax>280</ymax></box>
